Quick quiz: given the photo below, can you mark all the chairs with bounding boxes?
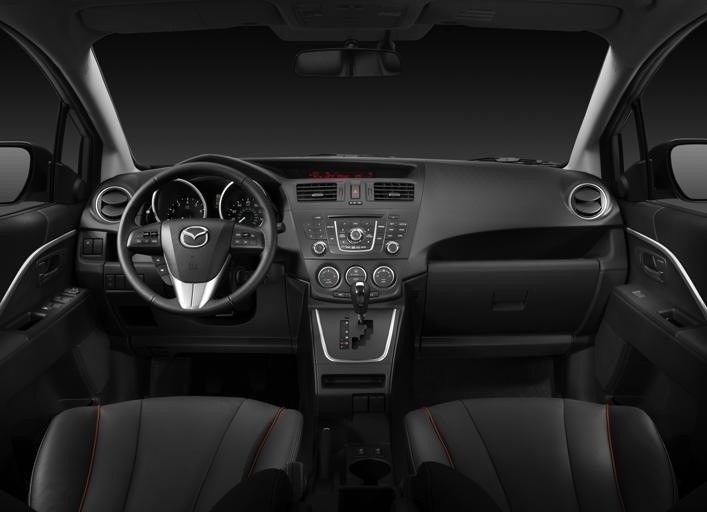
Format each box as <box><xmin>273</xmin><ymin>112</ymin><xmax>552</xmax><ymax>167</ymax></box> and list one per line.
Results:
<box><xmin>399</xmin><ymin>394</ymin><xmax>679</xmax><ymax>509</ymax></box>
<box><xmin>26</xmin><ymin>394</ymin><xmax>311</xmax><ymax>509</ymax></box>
